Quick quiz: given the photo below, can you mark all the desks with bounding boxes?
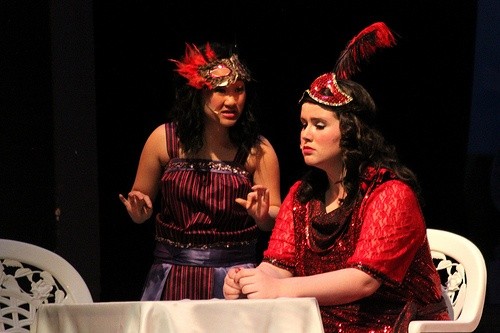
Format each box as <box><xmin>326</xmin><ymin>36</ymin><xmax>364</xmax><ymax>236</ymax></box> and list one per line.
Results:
<box><xmin>36</xmin><ymin>297</ymin><xmax>326</xmax><ymax>333</ymax></box>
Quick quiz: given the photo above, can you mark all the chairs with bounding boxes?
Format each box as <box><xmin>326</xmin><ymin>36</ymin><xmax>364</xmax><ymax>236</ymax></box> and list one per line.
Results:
<box><xmin>0</xmin><ymin>238</ymin><xmax>95</xmax><ymax>333</ymax></box>
<box><xmin>408</xmin><ymin>228</ymin><xmax>487</xmax><ymax>333</ymax></box>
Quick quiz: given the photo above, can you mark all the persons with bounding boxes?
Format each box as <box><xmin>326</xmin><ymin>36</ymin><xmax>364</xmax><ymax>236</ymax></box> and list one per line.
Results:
<box><xmin>222</xmin><ymin>73</ymin><xmax>452</xmax><ymax>333</ymax></box>
<box><xmin>118</xmin><ymin>43</ymin><xmax>282</xmax><ymax>300</ymax></box>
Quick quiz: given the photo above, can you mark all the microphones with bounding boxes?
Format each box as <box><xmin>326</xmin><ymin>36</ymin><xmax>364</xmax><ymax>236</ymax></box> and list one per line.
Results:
<box><xmin>205</xmin><ymin>101</ymin><xmax>218</xmax><ymax>116</ymax></box>
<box><xmin>298</xmin><ymin>145</ymin><xmax>302</xmax><ymax>149</ymax></box>
